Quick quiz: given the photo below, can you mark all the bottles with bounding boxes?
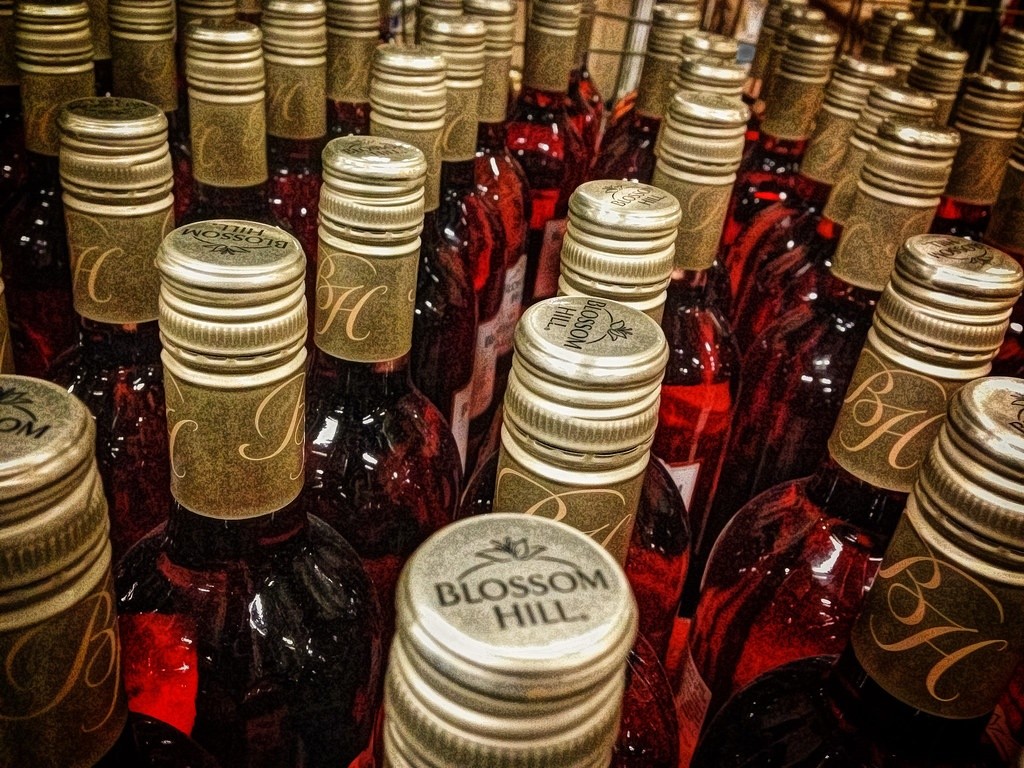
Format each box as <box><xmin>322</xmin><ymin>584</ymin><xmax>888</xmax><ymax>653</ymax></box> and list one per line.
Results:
<box><xmin>0</xmin><ymin>0</ymin><xmax>1024</xmax><ymax>768</ymax></box>
